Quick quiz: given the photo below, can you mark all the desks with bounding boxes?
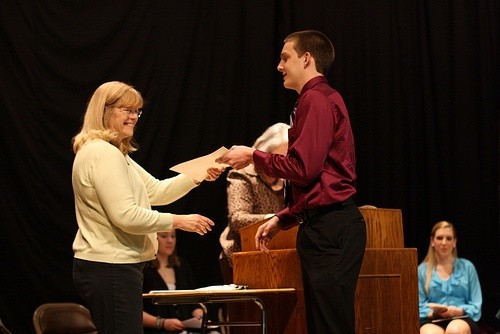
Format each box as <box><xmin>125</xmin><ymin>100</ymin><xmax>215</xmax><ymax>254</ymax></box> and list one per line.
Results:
<box><xmin>144</xmin><ymin>289</ymin><xmax>296</xmax><ymax>334</ymax></box>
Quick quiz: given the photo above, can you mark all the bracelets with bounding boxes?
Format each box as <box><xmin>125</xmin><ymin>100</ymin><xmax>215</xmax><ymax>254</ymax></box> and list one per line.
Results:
<box><xmin>154</xmin><ymin>315</ymin><xmax>165</xmax><ymax>331</ymax></box>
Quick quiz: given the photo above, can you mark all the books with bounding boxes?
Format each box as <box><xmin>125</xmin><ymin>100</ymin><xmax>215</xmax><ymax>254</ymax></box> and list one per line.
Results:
<box><xmin>432</xmin><ymin>314</ymin><xmax>469</xmax><ymax>323</ymax></box>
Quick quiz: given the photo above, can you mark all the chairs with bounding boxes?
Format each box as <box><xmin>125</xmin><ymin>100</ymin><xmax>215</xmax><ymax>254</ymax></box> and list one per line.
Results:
<box><xmin>33</xmin><ymin>303</ymin><xmax>97</xmax><ymax>334</ymax></box>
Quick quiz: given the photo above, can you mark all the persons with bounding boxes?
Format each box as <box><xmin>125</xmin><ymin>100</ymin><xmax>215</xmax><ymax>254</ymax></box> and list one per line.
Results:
<box><xmin>215</xmin><ymin>28</ymin><xmax>367</xmax><ymax>333</ymax></box>
<box><xmin>417</xmin><ymin>221</ymin><xmax>483</xmax><ymax>333</ymax></box>
<box><xmin>220</xmin><ymin>123</ymin><xmax>292</xmax><ymax>331</ymax></box>
<box><xmin>72</xmin><ymin>81</ymin><xmax>225</xmax><ymax>333</ymax></box>
<box><xmin>143</xmin><ymin>229</ymin><xmax>220</xmax><ymax>333</ymax></box>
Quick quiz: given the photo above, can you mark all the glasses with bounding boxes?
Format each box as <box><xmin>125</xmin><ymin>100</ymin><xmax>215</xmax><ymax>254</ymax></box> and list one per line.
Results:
<box><xmin>109</xmin><ymin>106</ymin><xmax>142</xmax><ymax>118</ymax></box>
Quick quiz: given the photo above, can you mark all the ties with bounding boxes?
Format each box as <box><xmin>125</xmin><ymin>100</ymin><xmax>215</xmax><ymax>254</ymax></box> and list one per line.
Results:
<box><xmin>282</xmin><ymin>103</ymin><xmax>298</xmax><ymax>207</ymax></box>
